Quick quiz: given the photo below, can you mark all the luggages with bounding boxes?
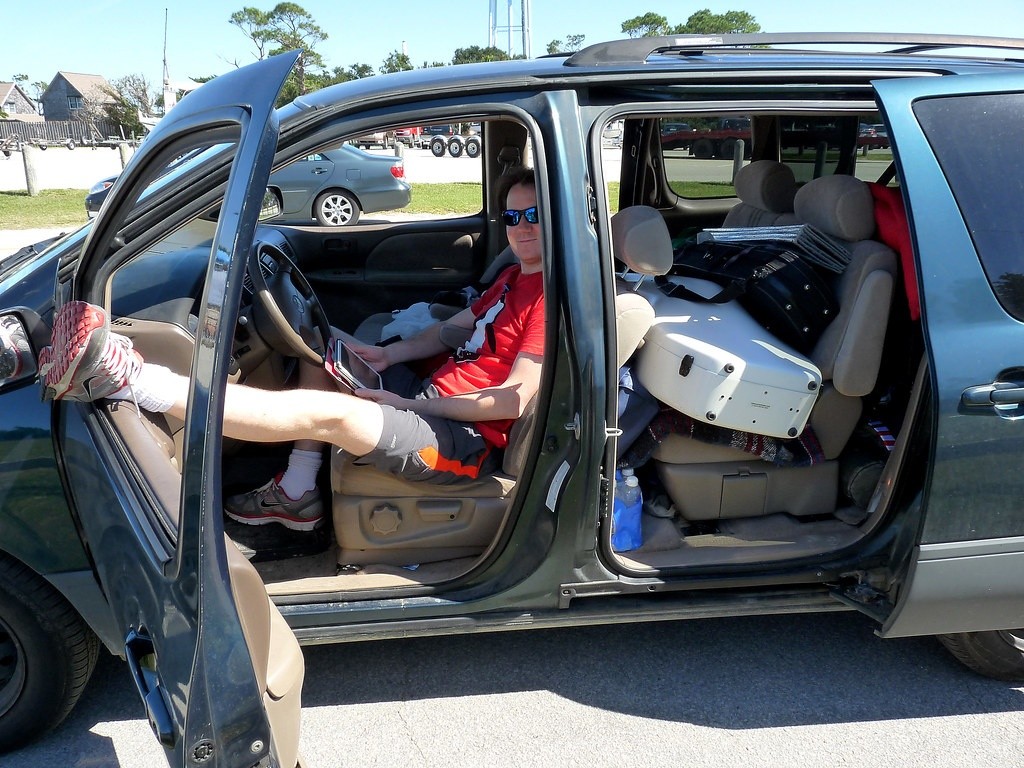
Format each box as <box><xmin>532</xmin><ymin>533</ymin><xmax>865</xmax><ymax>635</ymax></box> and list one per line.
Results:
<box><xmin>615</xmin><ymin>270</ymin><xmax>821</xmax><ymax>438</ymax></box>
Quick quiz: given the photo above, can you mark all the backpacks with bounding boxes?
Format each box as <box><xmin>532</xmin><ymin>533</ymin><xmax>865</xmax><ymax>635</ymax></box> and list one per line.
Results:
<box><xmin>656</xmin><ymin>240</ymin><xmax>841</xmax><ymax>355</ymax></box>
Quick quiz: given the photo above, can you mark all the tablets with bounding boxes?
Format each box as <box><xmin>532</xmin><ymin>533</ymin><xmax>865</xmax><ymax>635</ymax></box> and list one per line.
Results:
<box><xmin>336</xmin><ymin>339</ymin><xmax>383</xmax><ymax>391</ymax></box>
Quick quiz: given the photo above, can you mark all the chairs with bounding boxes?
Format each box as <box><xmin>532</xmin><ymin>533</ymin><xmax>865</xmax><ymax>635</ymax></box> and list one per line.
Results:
<box><xmin>650</xmin><ymin>175</ymin><xmax>899</xmax><ymax>527</ymax></box>
<box><xmin>352</xmin><ymin>246</ymin><xmax>522</xmax><ymax>362</ymax></box>
<box><xmin>327</xmin><ymin>206</ymin><xmax>672</xmax><ymax>565</ymax></box>
<box><xmin>721</xmin><ymin>159</ymin><xmax>802</xmax><ymax>231</ymax></box>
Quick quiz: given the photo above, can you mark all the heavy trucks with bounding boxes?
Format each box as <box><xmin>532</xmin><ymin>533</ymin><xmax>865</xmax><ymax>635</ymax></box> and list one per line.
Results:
<box><xmin>660</xmin><ymin>124</ymin><xmax>751</xmax><ymax>159</ymax></box>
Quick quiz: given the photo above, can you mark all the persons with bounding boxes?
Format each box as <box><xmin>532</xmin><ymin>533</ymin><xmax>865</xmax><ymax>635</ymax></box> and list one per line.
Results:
<box><xmin>38</xmin><ymin>169</ymin><xmax>555</xmax><ymax>528</ymax></box>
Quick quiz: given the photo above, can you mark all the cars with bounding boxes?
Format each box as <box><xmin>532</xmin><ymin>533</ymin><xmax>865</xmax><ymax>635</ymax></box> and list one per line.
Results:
<box><xmin>84</xmin><ymin>140</ymin><xmax>412</xmax><ymax>230</ymax></box>
<box><xmin>857</xmin><ymin>121</ymin><xmax>877</xmax><ymax>137</ymax></box>
<box><xmin>351</xmin><ymin>130</ymin><xmax>398</xmax><ymax>150</ymax></box>
<box><xmin>659</xmin><ymin>122</ymin><xmax>692</xmax><ymax>133</ymax></box>
<box><xmin>868</xmin><ymin>123</ymin><xmax>889</xmax><ymax>138</ymax></box>
<box><xmin>718</xmin><ymin>116</ymin><xmax>753</xmax><ymax>131</ymax></box>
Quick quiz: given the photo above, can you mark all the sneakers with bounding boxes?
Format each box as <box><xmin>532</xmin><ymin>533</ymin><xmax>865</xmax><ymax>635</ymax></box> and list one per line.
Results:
<box><xmin>37</xmin><ymin>300</ymin><xmax>144</xmax><ymax>404</ymax></box>
<box><xmin>224</xmin><ymin>474</ymin><xmax>325</xmax><ymax>530</ymax></box>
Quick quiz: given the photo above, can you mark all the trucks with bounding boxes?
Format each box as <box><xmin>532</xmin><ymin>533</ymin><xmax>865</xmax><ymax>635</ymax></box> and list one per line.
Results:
<box><xmin>394</xmin><ymin>125</ymin><xmax>423</xmax><ymax>148</ymax></box>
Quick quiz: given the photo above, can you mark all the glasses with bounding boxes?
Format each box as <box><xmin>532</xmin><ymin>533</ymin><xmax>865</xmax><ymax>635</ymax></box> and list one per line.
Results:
<box><xmin>502</xmin><ymin>205</ymin><xmax>539</xmax><ymax>226</ymax></box>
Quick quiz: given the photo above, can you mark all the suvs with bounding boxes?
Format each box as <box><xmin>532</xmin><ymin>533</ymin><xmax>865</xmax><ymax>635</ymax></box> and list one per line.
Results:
<box><xmin>0</xmin><ymin>27</ymin><xmax>1024</xmax><ymax>768</ymax></box>
